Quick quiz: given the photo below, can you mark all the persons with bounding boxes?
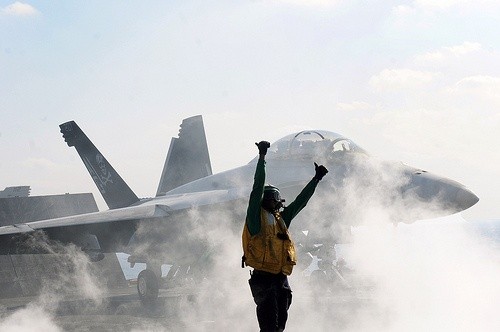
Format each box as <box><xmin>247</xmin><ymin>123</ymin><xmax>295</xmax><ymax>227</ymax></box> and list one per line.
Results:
<box><xmin>241</xmin><ymin>140</ymin><xmax>328</xmax><ymax>332</ymax></box>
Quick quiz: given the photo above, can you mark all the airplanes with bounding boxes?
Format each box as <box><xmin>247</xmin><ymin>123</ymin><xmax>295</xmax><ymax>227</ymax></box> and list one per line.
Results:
<box><xmin>0</xmin><ymin>114</ymin><xmax>479</xmax><ymax>303</ymax></box>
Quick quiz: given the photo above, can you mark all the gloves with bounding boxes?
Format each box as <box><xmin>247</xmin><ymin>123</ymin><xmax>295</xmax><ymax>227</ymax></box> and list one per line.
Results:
<box><xmin>314</xmin><ymin>162</ymin><xmax>328</xmax><ymax>180</ymax></box>
<box><xmin>255</xmin><ymin>140</ymin><xmax>271</xmax><ymax>155</ymax></box>
